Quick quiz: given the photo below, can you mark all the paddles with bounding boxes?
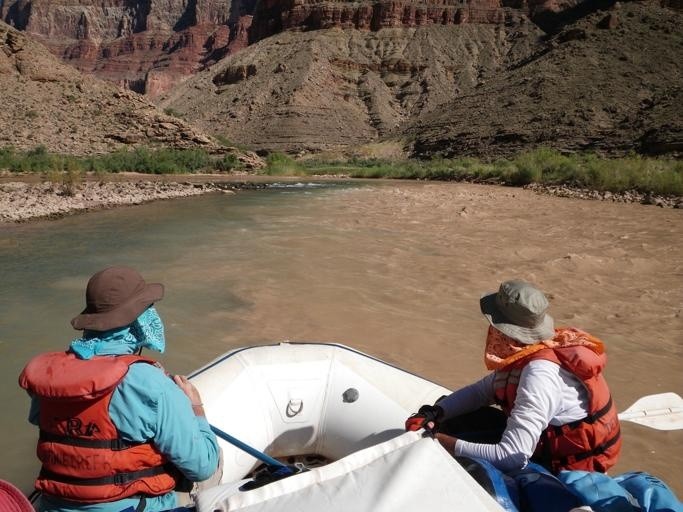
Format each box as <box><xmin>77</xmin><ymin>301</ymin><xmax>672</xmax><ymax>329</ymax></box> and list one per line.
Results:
<box><xmin>617</xmin><ymin>393</ymin><xmax>683</xmax><ymax>431</ymax></box>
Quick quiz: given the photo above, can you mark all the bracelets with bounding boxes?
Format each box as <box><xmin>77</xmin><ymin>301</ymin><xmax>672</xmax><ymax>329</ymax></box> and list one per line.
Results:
<box><xmin>191</xmin><ymin>403</ymin><xmax>204</xmax><ymax>407</ymax></box>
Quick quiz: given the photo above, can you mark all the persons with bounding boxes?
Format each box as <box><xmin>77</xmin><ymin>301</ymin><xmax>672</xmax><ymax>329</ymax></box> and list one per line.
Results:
<box><xmin>16</xmin><ymin>265</ymin><xmax>219</xmax><ymax>511</ymax></box>
<box><xmin>404</xmin><ymin>276</ymin><xmax>623</xmax><ymax>475</ymax></box>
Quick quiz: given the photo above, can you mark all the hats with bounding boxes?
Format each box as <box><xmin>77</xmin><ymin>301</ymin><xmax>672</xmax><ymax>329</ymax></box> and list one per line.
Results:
<box><xmin>478</xmin><ymin>280</ymin><xmax>557</xmax><ymax>346</ymax></box>
<box><xmin>71</xmin><ymin>266</ymin><xmax>165</xmax><ymax>334</ymax></box>
